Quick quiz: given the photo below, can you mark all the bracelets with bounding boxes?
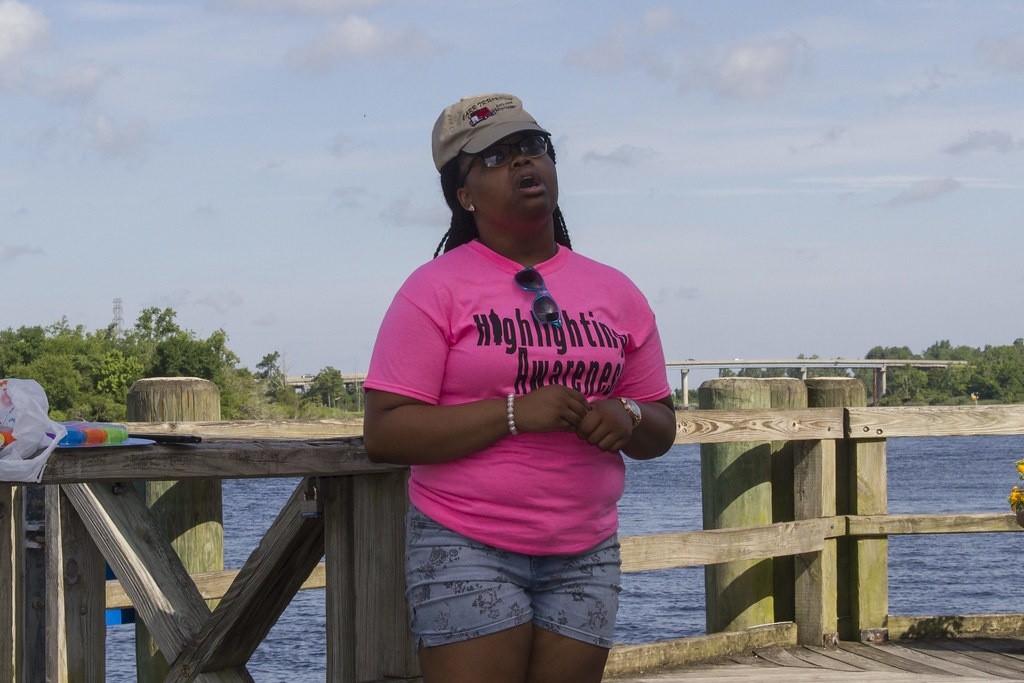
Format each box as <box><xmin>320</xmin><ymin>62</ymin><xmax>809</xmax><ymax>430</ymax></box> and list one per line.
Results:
<box><xmin>505</xmin><ymin>388</ymin><xmax>520</xmax><ymax>435</ymax></box>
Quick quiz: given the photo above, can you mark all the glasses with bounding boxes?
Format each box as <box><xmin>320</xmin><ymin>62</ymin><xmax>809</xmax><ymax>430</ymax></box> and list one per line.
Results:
<box><xmin>513</xmin><ymin>264</ymin><xmax>562</xmax><ymax>327</ymax></box>
<box><xmin>459</xmin><ymin>136</ymin><xmax>547</xmax><ymax>188</ymax></box>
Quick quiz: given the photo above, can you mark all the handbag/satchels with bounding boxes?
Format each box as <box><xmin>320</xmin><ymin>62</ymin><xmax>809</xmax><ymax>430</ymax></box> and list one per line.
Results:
<box><xmin>0</xmin><ymin>378</ymin><xmax>68</xmax><ymax>484</ymax></box>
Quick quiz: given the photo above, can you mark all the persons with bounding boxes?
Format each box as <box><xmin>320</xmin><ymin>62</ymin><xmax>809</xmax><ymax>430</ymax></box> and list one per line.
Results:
<box><xmin>357</xmin><ymin>86</ymin><xmax>680</xmax><ymax>683</ymax></box>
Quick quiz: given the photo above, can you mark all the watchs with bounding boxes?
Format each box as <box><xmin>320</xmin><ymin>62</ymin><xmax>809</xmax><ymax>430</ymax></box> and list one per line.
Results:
<box><xmin>617</xmin><ymin>395</ymin><xmax>644</xmax><ymax>428</ymax></box>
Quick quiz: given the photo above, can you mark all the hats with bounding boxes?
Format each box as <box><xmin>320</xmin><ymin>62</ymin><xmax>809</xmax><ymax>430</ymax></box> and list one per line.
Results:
<box><xmin>431</xmin><ymin>92</ymin><xmax>552</xmax><ymax>174</ymax></box>
